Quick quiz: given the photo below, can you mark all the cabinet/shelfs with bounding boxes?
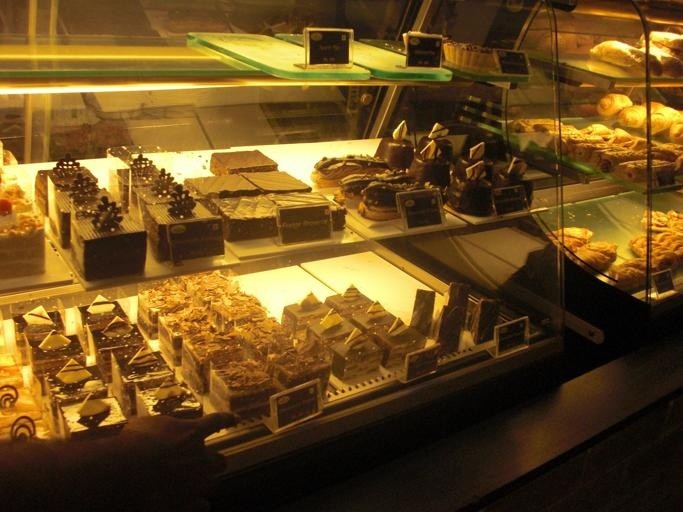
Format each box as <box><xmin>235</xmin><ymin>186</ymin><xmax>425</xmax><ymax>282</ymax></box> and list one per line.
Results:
<box><xmin>0</xmin><ymin>0</ymin><xmax>563</xmax><ymax>480</ymax></box>
<box><xmin>475</xmin><ymin>1</ymin><xmax>681</xmax><ymax>306</ymax></box>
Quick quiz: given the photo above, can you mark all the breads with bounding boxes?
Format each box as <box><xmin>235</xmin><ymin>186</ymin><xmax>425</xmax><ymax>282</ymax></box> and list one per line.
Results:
<box><xmin>510</xmin><ymin>30</ymin><xmax>683</xmax><ymax>291</ymax></box>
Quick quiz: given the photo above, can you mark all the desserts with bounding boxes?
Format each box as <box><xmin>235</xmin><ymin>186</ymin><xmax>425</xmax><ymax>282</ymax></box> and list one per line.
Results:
<box><xmin>0</xmin><ymin>268</ymin><xmax>531</xmax><ymax>442</ymax></box>
<box><xmin>0</xmin><ymin>120</ymin><xmax>531</xmax><ymax>280</ymax></box>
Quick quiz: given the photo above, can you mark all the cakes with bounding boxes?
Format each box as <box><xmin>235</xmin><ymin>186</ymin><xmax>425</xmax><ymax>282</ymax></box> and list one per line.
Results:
<box><xmin>443</xmin><ymin>39</ymin><xmax>498</xmax><ymax>72</ymax></box>
